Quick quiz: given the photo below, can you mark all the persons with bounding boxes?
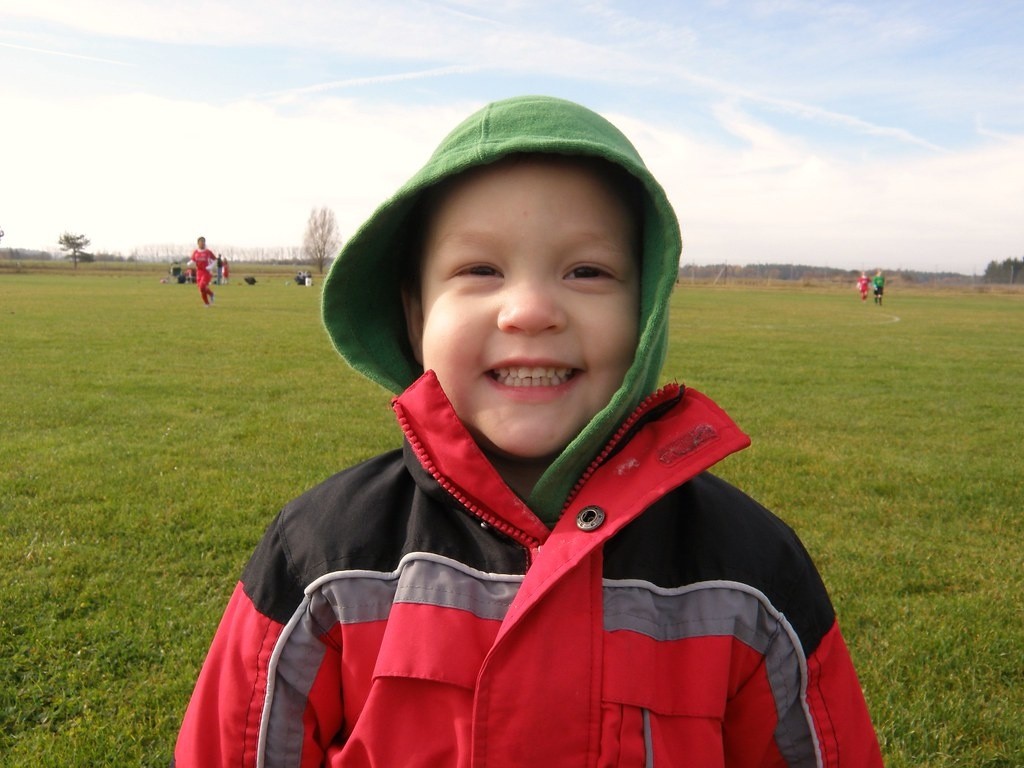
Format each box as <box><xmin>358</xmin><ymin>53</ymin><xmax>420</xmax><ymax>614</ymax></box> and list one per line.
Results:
<box><xmin>217</xmin><ymin>253</ymin><xmax>223</xmax><ymax>285</ymax></box>
<box><xmin>183</xmin><ymin>271</ymin><xmax>190</xmax><ymax>280</ymax></box>
<box><xmin>677</xmin><ymin>278</ymin><xmax>680</xmax><ymax>288</ymax></box>
<box><xmin>872</xmin><ymin>268</ymin><xmax>885</xmax><ymax>305</ymax></box>
<box><xmin>177</xmin><ymin>272</ymin><xmax>185</xmax><ymax>283</ymax></box>
<box><xmin>857</xmin><ymin>269</ymin><xmax>872</xmax><ymax>304</ymax></box>
<box><xmin>167</xmin><ymin>88</ymin><xmax>897</xmax><ymax>768</ymax></box>
<box><xmin>191</xmin><ymin>269</ymin><xmax>196</xmax><ymax>284</ymax></box>
<box><xmin>159</xmin><ymin>277</ymin><xmax>169</xmax><ymax>283</ymax></box>
<box><xmin>296</xmin><ymin>271</ymin><xmax>310</xmax><ymax>285</ymax></box>
<box><xmin>244</xmin><ymin>275</ymin><xmax>257</xmax><ymax>285</ymax></box>
<box><xmin>221</xmin><ymin>257</ymin><xmax>230</xmax><ymax>285</ymax></box>
<box><xmin>190</xmin><ymin>236</ymin><xmax>218</xmax><ymax>307</ymax></box>
